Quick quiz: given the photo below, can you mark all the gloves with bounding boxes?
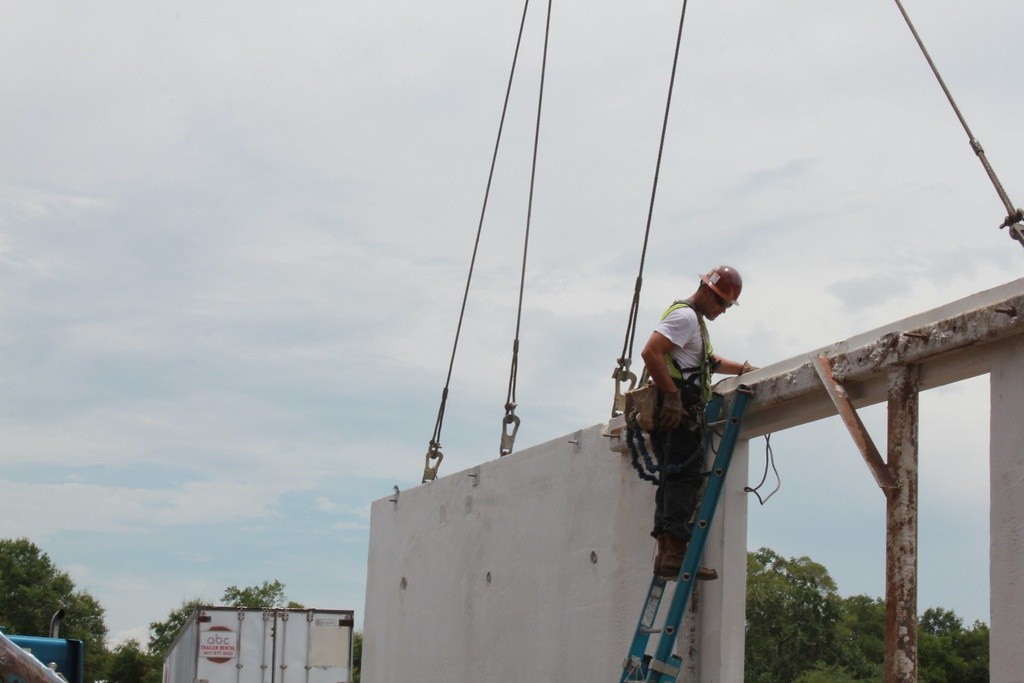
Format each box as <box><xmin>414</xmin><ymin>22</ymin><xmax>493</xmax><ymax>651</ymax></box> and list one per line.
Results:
<box><xmin>740</xmin><ymin>360</ymin><xmax>758</xmax><ymax>375</ymax></box>
<box><xmin>658</xmin><ymin>388</ymin><xmax>689</xmax><ymax>429</ymax></box>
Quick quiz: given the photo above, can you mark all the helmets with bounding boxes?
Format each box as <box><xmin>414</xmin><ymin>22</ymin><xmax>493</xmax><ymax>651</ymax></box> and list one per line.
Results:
<box><xmin>698</xmin><ymin>265</ymin><xmax>742</xmax><ymax>306</ymax></box>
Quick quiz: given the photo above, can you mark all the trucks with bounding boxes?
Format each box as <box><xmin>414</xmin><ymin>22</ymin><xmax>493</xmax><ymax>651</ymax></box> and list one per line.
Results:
<box><xmin>0</xmin><ymin>609</ymin><xmax>83</xmax><ymax>683</ymax></box>
<box><xmin>162</xmin><ymin>605</ymin><xmax>354</xmax><ymax>683</ymax></box>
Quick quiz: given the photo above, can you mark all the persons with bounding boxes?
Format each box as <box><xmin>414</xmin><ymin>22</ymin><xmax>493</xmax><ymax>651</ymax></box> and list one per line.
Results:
<box><xmin>641</xmin><ymin>264</ymin><xmax>759</xmax><ymax>580</ymax></box>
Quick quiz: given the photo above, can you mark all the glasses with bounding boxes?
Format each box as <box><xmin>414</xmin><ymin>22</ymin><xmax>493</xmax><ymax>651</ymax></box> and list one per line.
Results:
<box><xmin>709</xmin><ymin>288</ymin><xmax>732</xmax><ymax>308</ymax></box>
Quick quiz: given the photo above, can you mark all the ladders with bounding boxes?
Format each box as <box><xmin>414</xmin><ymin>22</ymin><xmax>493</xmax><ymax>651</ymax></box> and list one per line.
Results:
<box><xmin>617</xmin><ymin>382</ymin><xmax>755</xmax><ymax>683</ymax></box>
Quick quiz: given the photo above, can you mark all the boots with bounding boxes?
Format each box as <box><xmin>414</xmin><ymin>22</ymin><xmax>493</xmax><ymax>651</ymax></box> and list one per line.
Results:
<box><xmin>654</xmin><ymin>533</ymin><xmax>718</xmax><ymax>580</ymax></box>
